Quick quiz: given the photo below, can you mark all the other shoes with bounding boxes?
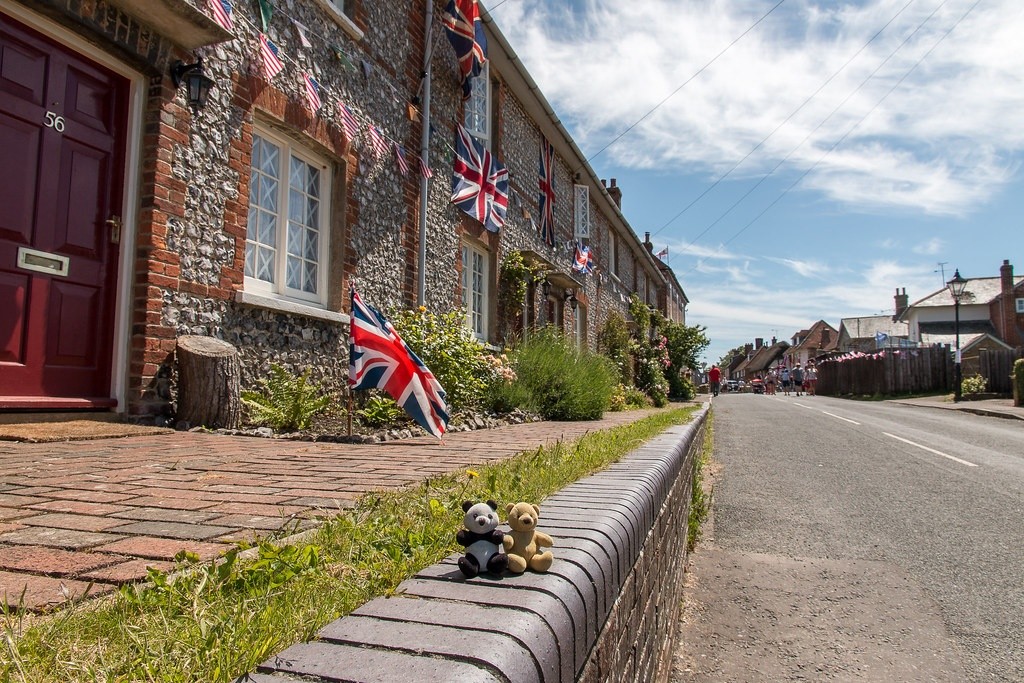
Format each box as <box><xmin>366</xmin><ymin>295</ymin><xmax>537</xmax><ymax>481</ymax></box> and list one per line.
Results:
<box><xmin>784</xmin><ymin>393</ymin><xmax>817</xmax><ymax>397</ymax></box>
<box><xmin>714</xmin><ymin>394</ymin><xmax>718</xmax><ymax>397</ymax></box>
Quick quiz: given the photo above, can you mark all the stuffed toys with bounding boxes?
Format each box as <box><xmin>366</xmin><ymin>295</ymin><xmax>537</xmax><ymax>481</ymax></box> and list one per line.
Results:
<box><xmin>502</xmin><ymin>502</ymin><xmax>553</xmax><ymax>572</ymax></box>
<box><xmin>457</xmin><ymin>500</ymin><xmax>509</xmax><ymax>576</ymax></box>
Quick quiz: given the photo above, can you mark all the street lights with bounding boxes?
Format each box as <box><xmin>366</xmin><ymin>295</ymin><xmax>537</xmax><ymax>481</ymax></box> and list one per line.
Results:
<box><xmin>947</xmin><ymin>269</ymin><xmax>969</xmax><ymax>401</ymax></box>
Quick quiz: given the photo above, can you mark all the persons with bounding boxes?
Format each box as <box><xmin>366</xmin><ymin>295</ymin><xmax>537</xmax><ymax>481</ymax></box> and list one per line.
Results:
<box><xmin>708</xmin><ymin>365</ymin><xmax>721</xmax><ymax>397</ymax></box>
<box><xmin>792</xmin><ymin>363</ymin><xmax>804</xmax><ymax>396</ymax></box>
<box><xmin>756</xmin><ymin>369</ymin><xmax>791</xmax><ymax>396</ymax></box>
<box><xmin>805</xmin><ymin>363</ymin><xmax>818</xmax><ymax>396</ymax></box>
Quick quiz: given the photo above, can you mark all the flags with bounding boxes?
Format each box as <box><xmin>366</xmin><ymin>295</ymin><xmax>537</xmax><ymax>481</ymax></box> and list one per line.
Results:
<box><xmin>208</xmin><ymin>0</ymin><xmax>233</xmax><ymax>29</ymax></box>
<box><xmin>449</xmin><ymin>123</ymin><xmax>509</xmax><ymax>234</ymax></box>
<box><xmin>416</xmin><ymin>157</ymin><xmax>433</xmax><ymax>179</ymax></box>
<box><xmin>259</xmin><ymin>33</ymin><xmax>285</xmax><ymax>84</ymax></box>
<box><xmin>367</xmin><ymin>124</ymin><xmax>389</xmax><ymax>159</ymax></box>
<box><xmin>258</xmin><ymin>0</ymin><xmax>274</xmax><ymax>33</ymax></box>
<box><xmin>536</xmin><ymin>133</ymin><xmax>557</xmax><ymax>248</ymax></box>
<box><xmin>295</xmin><ymin>24</ymin><xmax>312</xmax><ymax>47</ymax></box>
<box><xmin>573</xmin><ymin>242</ymin><xmax>593</xmax><ymax>277</ymax></box>
<box><xmin>362</xmin><ymin>60</ymin><xmax>371</xmax><ymax>79</ymax></box>
<box><xmin>303</xmin><ymin>73</ymin><xmax>322</xmax><ymax>118</ymax></box>
<box><xmin>408</xmin><ymin>105</ymin><xmax>420</xmax><ymax>122</ymax></box>
<box><xmin>337</xmin><ymin>101</ymin><xmax>361</xmax><ymax>144</ymax></box>
<box><xmin>332</xmin><ymin>46</ymin><xmax>355</xmax><ymax>71</ymax></box>
<box><xmin>656</xmin><ymin>247</ymin><xmax>668</xmax><ymax>258</ymax></box>
<box><xmin>347</xmin><ymin>283</ymin><xmax>451</xmax><ymax>439</ymax></box>
<box><xmin>394</xmin><ymin>143</ymin><xmax>409</xmax><ymax>177</ymax></box>
<box><xmin>441</xmin><ymin>0</ymin><xmax>489</xmax><ymax>101</ymax></box>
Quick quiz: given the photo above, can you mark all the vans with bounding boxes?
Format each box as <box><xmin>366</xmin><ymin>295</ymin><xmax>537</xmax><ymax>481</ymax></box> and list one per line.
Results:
<box><xmin>728</xmin><ymin>381</ymin><xmax>738</xmax><ymax>391</ymax></box>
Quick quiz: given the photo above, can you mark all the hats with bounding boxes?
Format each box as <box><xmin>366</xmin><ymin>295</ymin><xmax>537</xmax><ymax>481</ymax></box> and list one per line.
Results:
<box><xmin>810</xmin><ymin>363</ymin><xmax>814</xmax><ymax>365</ymax></box>
<box><xmin>795</xmin><ymin>363</ymin><xmax>801</xmax><ymax>367</ymax></box>
